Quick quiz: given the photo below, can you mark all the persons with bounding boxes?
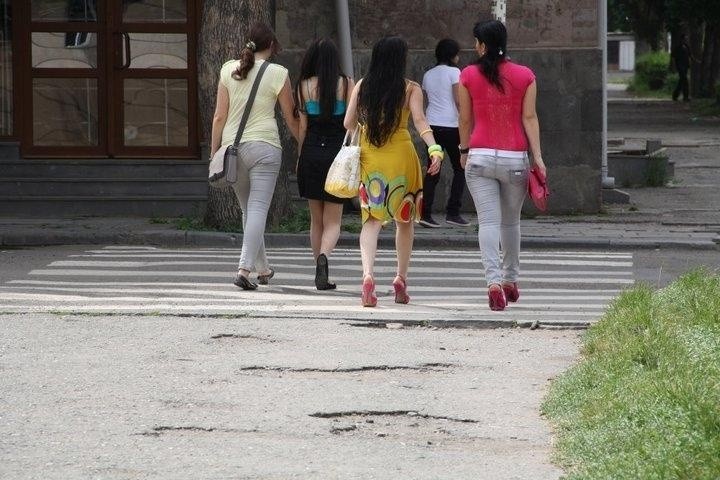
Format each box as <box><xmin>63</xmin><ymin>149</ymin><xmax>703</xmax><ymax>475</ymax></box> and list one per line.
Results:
<box><xmin>342</xmin><ymin>35</ymin><xmax>447</xmax><ymax>307</ymax></box>
<box><xmin>672</xmin><ymin>39</ymin><xmax>701</xmax><ymax>103</ymax></box>
<box><xmin>209</xmin><ymin>23</ymin><xmax>302</xmax><ymax>290</ymax></box>
<box><xmin>457</xmin><ymin>20</ymin><xmax>548</xmax><ymax>309</ymax></box>
<box><xmin>419</xmin><ymin>38</ymin><xmax>472</xmax><ymax>227</ymax></box>
<box><xmin>295</xmin><ymin>34</ymin><xmax>356</xmax><ymax>291</ymax></box>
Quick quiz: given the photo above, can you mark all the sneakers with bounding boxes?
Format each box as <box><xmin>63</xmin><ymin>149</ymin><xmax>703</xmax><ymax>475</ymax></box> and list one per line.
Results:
<box><xmin>445</xmin><ymin>215</ymin><xmax>472</xmax><ymax>227</ymax></box>
<box><xmin>314</xmin><ymin>254</ymin><xmax>336</xmax><ymax>290</ymax></box>
<box><xmin>418</xmin><ymin>216</ymin><xmax>442</xmax><ymax>229</ymax></box>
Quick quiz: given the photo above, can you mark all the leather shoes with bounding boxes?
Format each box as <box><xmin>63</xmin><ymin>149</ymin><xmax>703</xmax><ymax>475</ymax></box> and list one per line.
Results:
<box><xmin>233</xmin><ymin>275</ymin><xmax>258</xmax><ymax>290</ymax></box>
<box><xmin>257</xmin><ymin>267</ymin><xmax>275</xmax><ymax>285</ymax></box>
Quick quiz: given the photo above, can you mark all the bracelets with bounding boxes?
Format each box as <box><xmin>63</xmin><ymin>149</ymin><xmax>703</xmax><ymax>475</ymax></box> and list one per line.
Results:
<box><xmin>211</xmin><ymin>151</ymin><xmax>216</xmax><ymax>155</ymax></box>
<box><xmin>298</xmin><ymin>156</ymin><xmax>300</xmax><ymax>158</ymax></box>
<box><xmin>458</xmin><ymin>144</ymin><xmax>469</xmax><ymax>155</ymax></box>
<box><xmin>428</xmin><ymin>144</ymin><xmax>442</xmax><ymax>154</ymax></box>
<box><xmin>429</xmin><ymin>150</ymin><xmax>443</xmax><ymax>161</ymax></box>
<box><xmin>420</xmin><ymin>129</ymin><xmax>433</xmax><ymax>138</ymax></box>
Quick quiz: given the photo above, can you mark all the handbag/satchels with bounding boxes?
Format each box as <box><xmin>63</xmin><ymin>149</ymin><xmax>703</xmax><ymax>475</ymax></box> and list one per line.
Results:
<box><xmin>323</xmin><ymin>144</ymin><xmax>366</xmax><ymax>200</ymax></box>
<box><xmin>208</xmin><ymin>143</ymin><xmax>238</xmax><ymax>189</ymax></box>
<box><xmin>524</xmin><ymin>164</ymin><xmax>552</xmax><ymax>214</ymax></box>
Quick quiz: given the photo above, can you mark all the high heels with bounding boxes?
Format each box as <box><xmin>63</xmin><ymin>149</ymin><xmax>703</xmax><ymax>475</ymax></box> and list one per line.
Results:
<box><xmin>392</xmin><ymin>275</ymin><xmax>411</xmax><ymax>305</ymax></box>
<box><xmin>487</xmin><ymin>280</ymin><xmax>522</xmax><ymax>311</ymax></box>
<box><xmin>360</xmin><ymin>273</ymin><xmax>379</xmax><ymax>307</ymax></box>
